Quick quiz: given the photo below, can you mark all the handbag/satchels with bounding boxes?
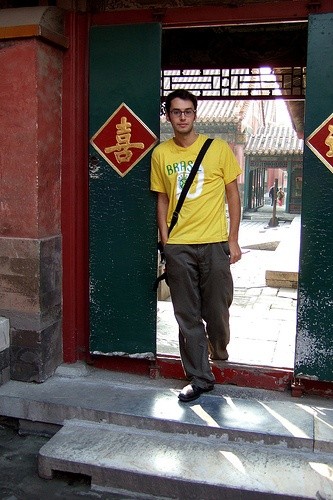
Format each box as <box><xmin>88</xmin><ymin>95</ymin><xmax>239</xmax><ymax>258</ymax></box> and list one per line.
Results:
<box><xmin>157</xmin><ymin>241</ymin><xmax>169</xmax><ymax>285</ymax></box>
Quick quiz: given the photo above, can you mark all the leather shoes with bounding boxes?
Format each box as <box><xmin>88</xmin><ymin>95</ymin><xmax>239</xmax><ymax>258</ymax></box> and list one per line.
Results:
<box><xmin>177</xmin><ymin>383</ymin><xmax>214</xmax><ymax>402</ymax></box>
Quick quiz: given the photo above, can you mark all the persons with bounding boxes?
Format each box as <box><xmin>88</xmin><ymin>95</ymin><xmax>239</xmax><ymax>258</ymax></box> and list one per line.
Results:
<box><xmin>268</xmin><ymin>183</ymin><xmax>278</xmax><ymax>206</ymax></box>
<box><xmin>276</xmin><ymin>188</ymin><xmax>284</xmax><ymax>206</ymax></box>
<box><xmin>150</xmin><ymin>90</ymin><xmax>241</xmax><ymax>402</ymax></box>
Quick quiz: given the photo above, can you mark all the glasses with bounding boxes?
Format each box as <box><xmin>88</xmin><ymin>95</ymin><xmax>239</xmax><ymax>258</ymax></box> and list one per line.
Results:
<box><xmin>170</xmin><ymin>109</ymin><xmax>197</xmax><ymax>117</ymax></box>
